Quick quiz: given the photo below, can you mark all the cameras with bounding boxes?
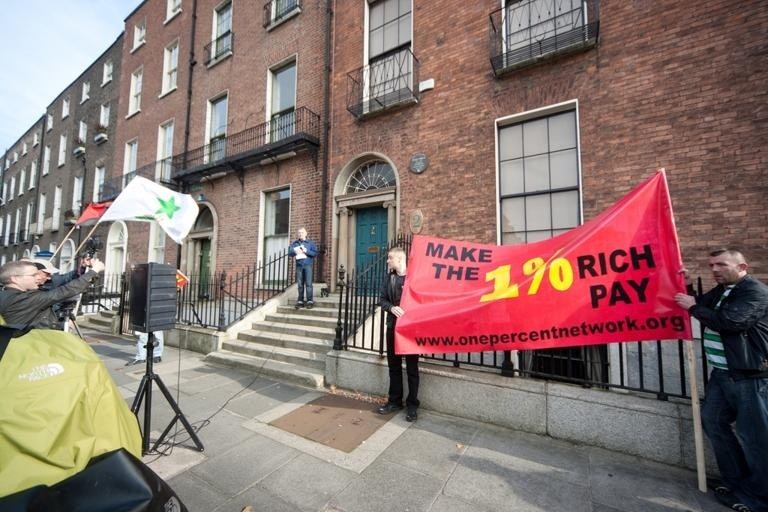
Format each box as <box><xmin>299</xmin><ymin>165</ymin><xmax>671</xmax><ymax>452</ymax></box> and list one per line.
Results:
<box><xmin>74</xmin><ymin>238</ymin><xmax>104</xmax><ymax>261</ymax></box>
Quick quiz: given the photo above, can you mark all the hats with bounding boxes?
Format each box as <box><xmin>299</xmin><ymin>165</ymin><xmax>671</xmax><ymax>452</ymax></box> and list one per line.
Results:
<box><xmin>33</xmin><ymin>260</ymin><xmax>59</xmax><ymax>273</ymax></box>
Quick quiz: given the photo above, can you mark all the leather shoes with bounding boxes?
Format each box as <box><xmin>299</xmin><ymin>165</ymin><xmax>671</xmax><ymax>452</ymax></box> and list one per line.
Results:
<box><xmin>378</xmin><ymin>403</ymin><xmax>404</xmax><ymax>414</ymax></box>
<box><xmin>406</xmin><ymin>410</ymin><xmax>417</xmax><ymax>422</ymax></box>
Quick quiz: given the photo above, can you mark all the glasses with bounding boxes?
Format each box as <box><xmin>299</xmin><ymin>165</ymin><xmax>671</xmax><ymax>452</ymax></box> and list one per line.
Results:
<box><xmin>14</xmin><ymin>272</ymin><xmax>39</xmax><ymax>277</ymax></box>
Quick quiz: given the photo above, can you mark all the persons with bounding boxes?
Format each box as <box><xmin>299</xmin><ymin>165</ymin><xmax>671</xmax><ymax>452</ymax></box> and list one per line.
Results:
<box><xmin>376</xmin><ymin>246</ymin><xmax>419</xmax><ymax>421</ymax></box>
<box><xmin>0</xmin><ymin>257</ymin><xmax>106</xmax><ymax>333</ymax></box>
<box><xmin>674</xmin><ymin>248</ymin><xmax>768</xmax><ymax>512</ymax></box>
<box><xmin>285</xmin><ymin>226</ymin><xmax>319</xmax><ymax>309</ymax></box>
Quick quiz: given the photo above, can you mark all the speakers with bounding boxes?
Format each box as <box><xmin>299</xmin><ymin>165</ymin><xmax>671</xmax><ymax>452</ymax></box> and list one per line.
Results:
<box><xmin>128</xmin><ymin>262</ymin><xmax>177</xmax><ymax>333</ymax></box>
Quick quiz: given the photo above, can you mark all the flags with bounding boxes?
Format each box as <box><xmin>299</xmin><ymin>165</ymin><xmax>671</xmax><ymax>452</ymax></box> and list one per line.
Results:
<box><xmin>98</xmin><ymin>173</ymin><xmax>200</xmax><ymax>245</ymax></box>
<box><xmin>76</xmin><ymin>200</ymin><xmax>114</xmax><ymax>225</ymax></box>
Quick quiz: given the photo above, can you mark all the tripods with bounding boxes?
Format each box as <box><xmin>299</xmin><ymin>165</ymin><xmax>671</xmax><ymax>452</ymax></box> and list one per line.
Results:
<box><xmin>129</xmin><ymin>333</ymin><xmax>204</xmax><ymax>456</ymax></box>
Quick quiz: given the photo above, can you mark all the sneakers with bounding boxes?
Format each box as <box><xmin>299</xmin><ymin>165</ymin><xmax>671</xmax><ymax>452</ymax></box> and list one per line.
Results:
<box><xmin>707</xmin><ymin>479</ymin><xmax>753</xmax><ymax>512</ymax></box>
<box><xmin>295</xmin><ymin>304</ymin><xmax>313</xmax><ymax>309</ymax></box>
